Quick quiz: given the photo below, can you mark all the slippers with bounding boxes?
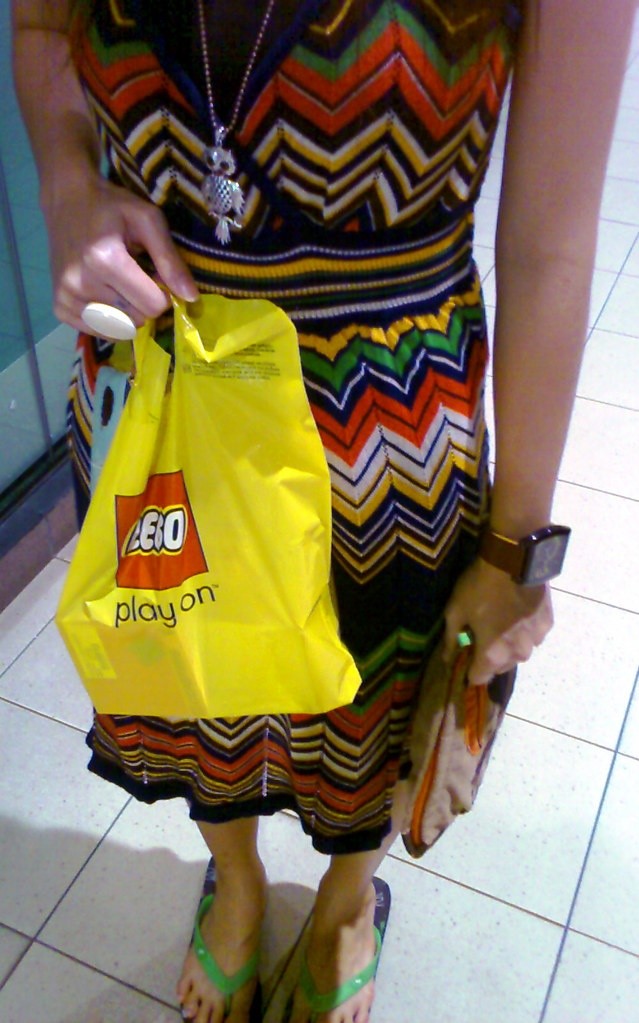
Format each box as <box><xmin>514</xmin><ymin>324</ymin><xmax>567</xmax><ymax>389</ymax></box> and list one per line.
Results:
<box><xmin>279</xmin><ymin>875</ymin><xmax>391</xmax><ymax>1023</ymax></box>
<box><xmin>180</xmin><ymin>855</ymin><xmax>263</xmax><ymax>1023</ymax></box>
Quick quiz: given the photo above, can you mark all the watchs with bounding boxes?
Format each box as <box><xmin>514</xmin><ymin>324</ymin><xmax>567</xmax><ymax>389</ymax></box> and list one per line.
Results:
<box><xmin>476</xmin><ymin>525</ymin><xmax>571</xmax><ymax>585</ymax></box>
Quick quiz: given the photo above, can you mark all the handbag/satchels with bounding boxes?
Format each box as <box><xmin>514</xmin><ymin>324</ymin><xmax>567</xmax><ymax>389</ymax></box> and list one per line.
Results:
<box><xmin>390</xmin><ymin>645</ymin><xmax>518</xmax><ymax>860</ymax></box>
<box><xmin>58</xmin><ymin>284</ymin><xmax>363</xmax><ymax>722</ymax></box>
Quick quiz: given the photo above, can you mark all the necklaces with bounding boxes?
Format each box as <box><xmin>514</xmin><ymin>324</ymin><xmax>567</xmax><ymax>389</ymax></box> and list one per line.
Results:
<box><xmin>198</xmin><ymin>0</ymin><xmax>275</xmax><ymax>245</ymax></box>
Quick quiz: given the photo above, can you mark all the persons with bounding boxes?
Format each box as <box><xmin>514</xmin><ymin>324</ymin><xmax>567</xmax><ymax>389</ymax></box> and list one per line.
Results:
<box><xmin>11</xmin><ymin>1</ymin><xmax>637</xmax><ymax>1023</ymax></box>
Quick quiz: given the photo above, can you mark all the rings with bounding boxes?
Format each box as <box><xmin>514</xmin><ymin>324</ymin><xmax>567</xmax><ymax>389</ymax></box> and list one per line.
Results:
<box><xmin>80</xmin><ymin>301</ymin><xmax>137</xmax><ymax>341</ymax></box>
